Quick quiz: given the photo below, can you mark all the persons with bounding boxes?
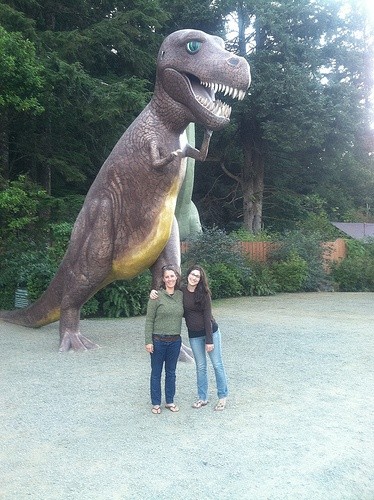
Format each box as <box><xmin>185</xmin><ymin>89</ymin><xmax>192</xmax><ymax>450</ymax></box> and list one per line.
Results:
<box><xmin>149</xmin><ymin>265</ymin><xmax>228</xmax><ymax>411</ymax></box>
<box><xmin>145</xmin><ymin>264</ymin><xmax>184</xmax><ymax>413</ymax></box>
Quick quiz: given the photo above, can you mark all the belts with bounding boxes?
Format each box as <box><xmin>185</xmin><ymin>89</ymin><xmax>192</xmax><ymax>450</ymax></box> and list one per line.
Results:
<box><xmin>153</xmin><ymin>335</ymin><xmax>180</xmax><ymax>342</ymax></box>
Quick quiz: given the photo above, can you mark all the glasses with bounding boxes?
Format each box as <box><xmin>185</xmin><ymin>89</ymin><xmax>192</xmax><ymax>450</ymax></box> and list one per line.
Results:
<box><xmin>161</xmin><ymin>264</ymin><xmax>174</xmax><ymax>270</ymax></box>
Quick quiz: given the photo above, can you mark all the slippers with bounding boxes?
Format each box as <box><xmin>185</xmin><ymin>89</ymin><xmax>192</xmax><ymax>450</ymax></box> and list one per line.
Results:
<box><xmin>151</xmin><ymin>407</ymin><xmax>161</xmax><ymax>414</ymax></box>
<box><xmin>165</xmin><ymin>405</ymin><xmax>179</xmax><ymax>412</ymax></box>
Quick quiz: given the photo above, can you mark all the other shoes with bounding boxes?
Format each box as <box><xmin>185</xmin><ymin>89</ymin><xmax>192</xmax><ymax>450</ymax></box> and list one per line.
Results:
<box><xmin>192</xmin><ymin>400</ymin><xmax>210</xmax><ymax>409</ymax></box>
<box><xmin>214</xmin><ymin>401</ymin><xmax>228</xmax><ymax>411</ymax></box>
